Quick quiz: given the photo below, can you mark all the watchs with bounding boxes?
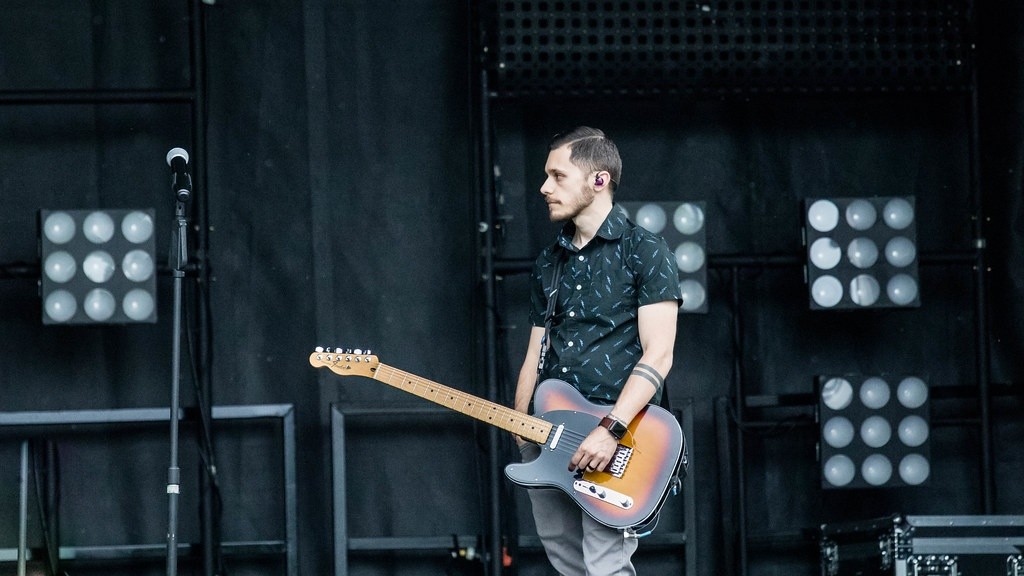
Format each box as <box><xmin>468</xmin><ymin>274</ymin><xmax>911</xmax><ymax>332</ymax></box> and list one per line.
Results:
<box><xmin>598</xmin><ymin>417</ymin><xmax>628</xmax><ymax>440</ymax></box>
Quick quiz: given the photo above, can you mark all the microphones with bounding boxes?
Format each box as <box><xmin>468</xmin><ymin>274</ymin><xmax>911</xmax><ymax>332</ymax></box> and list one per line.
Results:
<box><xmin>166</xmin><ymin>147</ymin><xmax>191</xmax><ymax>202</ymax></box>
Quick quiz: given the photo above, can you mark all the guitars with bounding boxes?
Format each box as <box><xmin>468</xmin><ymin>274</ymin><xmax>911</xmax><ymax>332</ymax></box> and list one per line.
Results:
<box><xmin>308</xmin><ymin>346</ymin><xmax>690</xmax><ymax>537</ymax></box>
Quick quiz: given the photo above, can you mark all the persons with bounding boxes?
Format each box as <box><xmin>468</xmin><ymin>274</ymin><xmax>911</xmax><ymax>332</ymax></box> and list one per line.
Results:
<box><xmin>513</xmin><ymin>127</ymin><xmax>684</xmax><ymax>576</ymax></box>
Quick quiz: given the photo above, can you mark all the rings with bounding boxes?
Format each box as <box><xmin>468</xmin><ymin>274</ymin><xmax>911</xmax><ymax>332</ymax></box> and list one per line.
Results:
<box><xmin>588</xmin><ymin>464</ymin><xmax>594</xmax><ymax>470</ymax></box>
